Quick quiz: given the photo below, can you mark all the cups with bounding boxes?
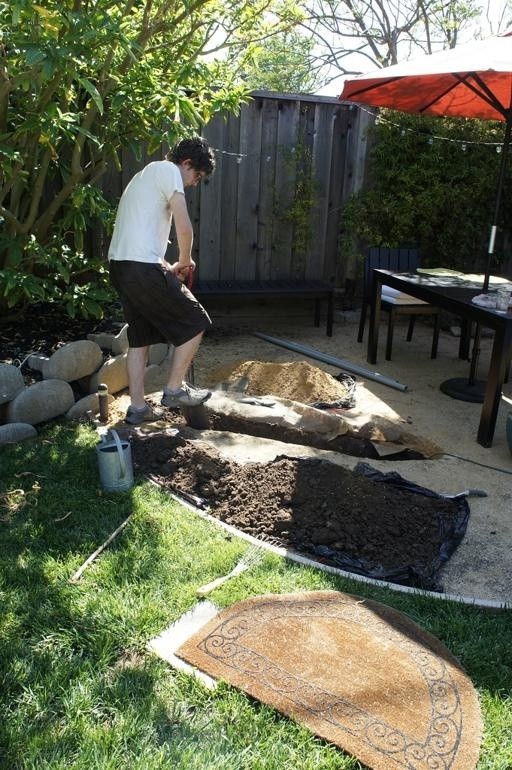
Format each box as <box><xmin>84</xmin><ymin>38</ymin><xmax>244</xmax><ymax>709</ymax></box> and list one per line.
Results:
<box><xmin>493</xmin><ymin>287</ymin><xmax>511</xmax><ymax>314</ymax></box>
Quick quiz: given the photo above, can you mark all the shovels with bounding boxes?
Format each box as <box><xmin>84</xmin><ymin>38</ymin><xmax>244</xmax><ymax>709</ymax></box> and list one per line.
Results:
<box><xmin>176</xmin><ymin>265</ymin><xmax>210</xmax><ymax>429</ymax></box>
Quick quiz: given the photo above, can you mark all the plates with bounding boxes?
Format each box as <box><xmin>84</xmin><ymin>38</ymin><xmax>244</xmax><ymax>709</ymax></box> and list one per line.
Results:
<box><xmin>416</xmin><ymin>266</ymin><xmax>463</xmax><ymax>279</ymax></box>
<box><xmin>459</xmin><ymin>272</ymin><xmax>508</xmax><ymax>285</ymax></box>
<box><xmin>471</xmin><ymin>293</ymin><xmax>512</xmax><ymax>310</ymax></box>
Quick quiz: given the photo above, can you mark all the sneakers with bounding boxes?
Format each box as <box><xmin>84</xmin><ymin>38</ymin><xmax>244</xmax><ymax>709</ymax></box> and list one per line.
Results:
<box><xmin>125</xmin><ymin>400</ymin><xmax>164</xmax><ymax>425</ymax></box>
<box><xmin>161</xmin><ymin>383</ymin><xmax>213</xmax><ymax>407</ymax></box>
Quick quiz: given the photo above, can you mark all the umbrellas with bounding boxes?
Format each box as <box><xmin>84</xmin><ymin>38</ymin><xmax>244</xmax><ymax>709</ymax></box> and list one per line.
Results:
<box><xmin>339</xmin><ymin>24</ymin><xmax>512</xmax><ymax>386</ymax></box>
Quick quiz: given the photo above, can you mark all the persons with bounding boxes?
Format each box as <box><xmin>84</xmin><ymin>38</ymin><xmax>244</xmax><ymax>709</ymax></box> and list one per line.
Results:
<box><xmin>108</xmin><ymin>137</ymin><xmax>212</xmax><ymax>425</ymax></box>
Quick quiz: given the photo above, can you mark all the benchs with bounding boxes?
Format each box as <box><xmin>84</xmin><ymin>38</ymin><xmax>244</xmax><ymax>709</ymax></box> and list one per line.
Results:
<box><xmin>182</xmin><ymin>277</ymin><xmax>335</xmax><ymax>338</ymax></box>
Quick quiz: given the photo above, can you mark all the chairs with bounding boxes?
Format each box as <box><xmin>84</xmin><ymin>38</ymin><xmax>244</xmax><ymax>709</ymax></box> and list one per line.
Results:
<box><xmin>357</xmin><ymin>246</ymin><xmax>442</xmax><ymax>361</ymax></box>
<box><xmin>367</xmin><ymin>263</ymin><xmax>512</xmax><ymax>448</ymax></box>
